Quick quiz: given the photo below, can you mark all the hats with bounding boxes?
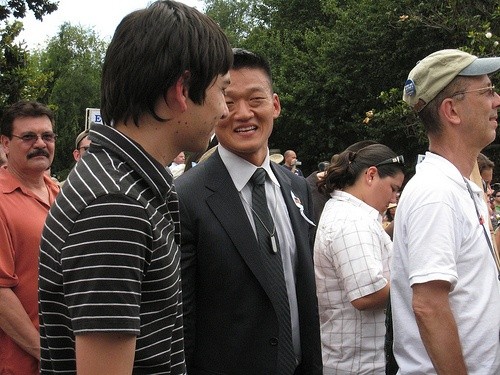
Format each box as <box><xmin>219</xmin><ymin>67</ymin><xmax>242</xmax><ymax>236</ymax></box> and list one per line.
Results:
<box><xmin>76</xmin><ymin>131</ymin><xmax>90</xmax><ymax>148</ymax></box>
<box><xmin>403</xmin><ymin>49</ymin><xmax>500</xmax><ymax>114</ymax></box>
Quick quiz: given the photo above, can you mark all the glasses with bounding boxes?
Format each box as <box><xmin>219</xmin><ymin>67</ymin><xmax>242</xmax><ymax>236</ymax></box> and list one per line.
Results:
<box><xmin>11</xmin><ymin>133</ymin><xmax>58</xmax><ymax>144</ymax></box>
<box><xmin>232</xmin><ymin>48</ymin><xmax>260</xmax><ymax>59</ymax></box>
<box><xmin>440</xmin><ymin>84</ymin><xmax>495</xmax><ymax>106</ymax></box>
<box><xmin>366</xmin><ymin>155</ymin><xmax>404</xmax><ymax>174</ymax></box>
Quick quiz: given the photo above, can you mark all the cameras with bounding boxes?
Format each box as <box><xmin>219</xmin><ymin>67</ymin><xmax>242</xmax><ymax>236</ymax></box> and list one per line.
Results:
<box><xmin>295</xmin><ymin>160</ymin><xmax>302</xmax><ymax>165</ymax></box>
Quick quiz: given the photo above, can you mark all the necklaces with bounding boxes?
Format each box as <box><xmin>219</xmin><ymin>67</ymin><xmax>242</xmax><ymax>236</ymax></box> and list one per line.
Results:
<box><xmin>237</xmin><ymin>183</ymin><xmax>283</xmax><ymax>254</ymax></box>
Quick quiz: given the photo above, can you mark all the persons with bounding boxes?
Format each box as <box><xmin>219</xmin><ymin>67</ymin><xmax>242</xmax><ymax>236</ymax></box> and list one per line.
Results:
<box><xmin>37</xmin><ymin>0</ymin><xmax>235</xmax><ymax>375</ymax></box>
<box><xmin>0</xmin><ymin>100</ymin><xmax>500</xmax><ymax>375</ymax></box>
<box><xmin>313</xmin><ymin>143</ymin><xmax>405</xmax><ymax>375</ymax></box>
<box><xmin>389</xmin><ymin>50</ymin><xmax>500</xmax><ymax>374</ymax></box>
<box><xmin>173</xmin><ymin>48</ymin><xmax>323</xmax><ymax>375</ymax></box>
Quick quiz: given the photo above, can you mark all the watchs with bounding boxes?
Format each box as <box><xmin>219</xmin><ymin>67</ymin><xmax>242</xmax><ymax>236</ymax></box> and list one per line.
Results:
<box><xmin>490</xmin><ymin>195</ymin><xmax>494</xmax><ymax>203</ymax></box>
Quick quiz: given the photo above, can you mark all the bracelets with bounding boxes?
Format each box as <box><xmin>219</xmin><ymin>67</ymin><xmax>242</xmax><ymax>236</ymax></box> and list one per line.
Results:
<box><xmin>491</xmin><ymin>207</ymin><xmax>496</xmax><ymax>210</ymax></box>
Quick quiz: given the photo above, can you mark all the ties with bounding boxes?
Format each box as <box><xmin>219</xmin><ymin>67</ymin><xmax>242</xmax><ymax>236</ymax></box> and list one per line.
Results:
<box><xmin>251</xmin><ymin>168</ymin><xmax>296</xmax><ymax>375</ymax></box>
